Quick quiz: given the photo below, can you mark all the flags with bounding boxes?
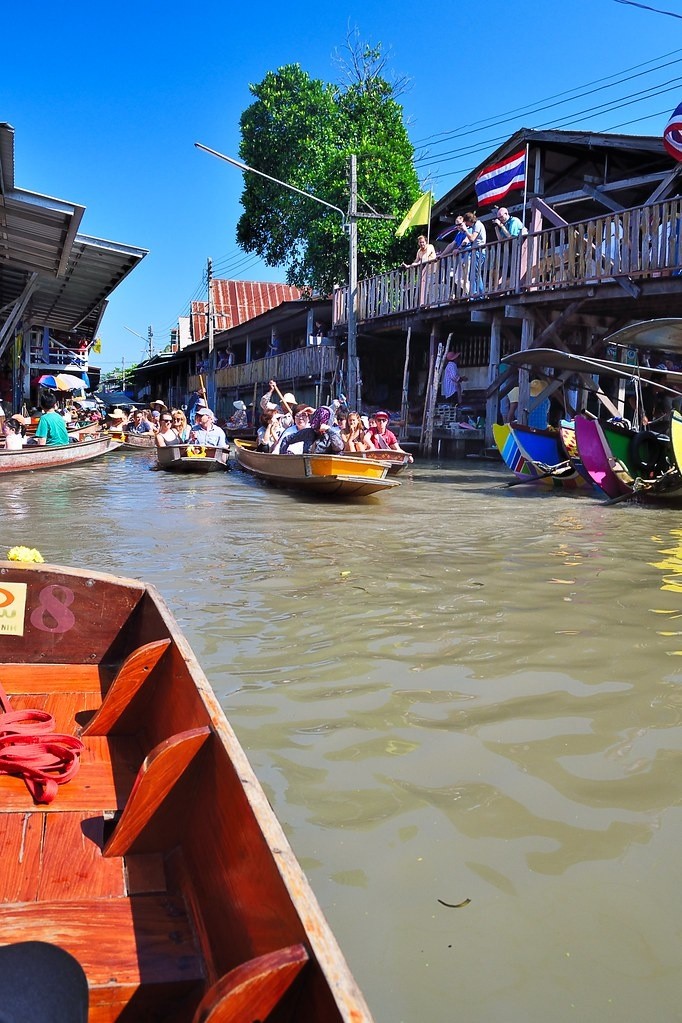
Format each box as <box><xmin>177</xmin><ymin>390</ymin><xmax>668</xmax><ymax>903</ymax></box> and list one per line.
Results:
<box><xmin>395</xmin><ymin>190</ymin><xmax>431</xmax><ymax>236</ymax></box>
<box><xmin>663</xmin><ymin>102</ymin><xmax>682</xmax><ymax>162</ymax></box>
<box><xmin>475</xmin><ymin>150</ymin><xmax>526</xmax><ymax>206</ymax></box>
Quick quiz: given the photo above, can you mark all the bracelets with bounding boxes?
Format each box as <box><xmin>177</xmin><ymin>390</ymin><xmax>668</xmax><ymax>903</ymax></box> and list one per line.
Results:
<box><xmin>458</xmin><ymin>247</ymin><xmax>461</xmax><ymax>250</ymax></box>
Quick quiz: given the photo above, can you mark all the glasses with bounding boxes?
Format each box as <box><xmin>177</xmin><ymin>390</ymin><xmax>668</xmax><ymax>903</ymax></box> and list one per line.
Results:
<box><xmin>337</xmin><ymin>417</ymin><xmax>345</xmax><ymax>421</ymax></box>
<box><xmin>163</xmin><ymin>420</ymin><xmax>173</xmax><ymax>424</ymax></box>
<box><xmin>173</xmin><ymin>418</ymin><xmax>181</xmax><ymax>422</ymax></box>
<box><xmin>377</xmin><ymin>418</ymin><xmax>387</xmax><ymax>422</ymax></box>
<box><xmin>294</xmin><ymin>413</ymin><xmax>306</xmax><ymax>418</ymax></box>
<box><xmin>456</xmin><ymin>223</ymin><xmax>462</xmax><ymax>226</ymax></box>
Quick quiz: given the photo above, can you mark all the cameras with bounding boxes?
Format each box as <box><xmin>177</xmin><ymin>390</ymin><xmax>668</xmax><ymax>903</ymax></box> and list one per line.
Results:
<box><xmin>492</xmin><ymin>217</ymin><xmax>500</xmax><ymax>227</ymax></box>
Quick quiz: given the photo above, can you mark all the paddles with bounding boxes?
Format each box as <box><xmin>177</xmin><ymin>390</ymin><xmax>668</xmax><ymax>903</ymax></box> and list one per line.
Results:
<box><xmin>273</xmin><ymin>382</ymin><xmax>293</xmax><ymax>413</ymax></box>
<box><xmin>341</xmin><ymin>395</ymin><xmax>352</xmax><ymax>411</ymax></box>
<box><xmin>495</xmin><ymin>465</ymin><xmax>572</xmax><ymax>488</ymax></box>
<box><xmin>198</xmin><ymin>375</ymin><xmax>210</xmax><ymax>408</ymax></box>
<box><xmin>599</xmin><ymin>474</ymin><xmax>678</xmax><ymax>505</ymax></box>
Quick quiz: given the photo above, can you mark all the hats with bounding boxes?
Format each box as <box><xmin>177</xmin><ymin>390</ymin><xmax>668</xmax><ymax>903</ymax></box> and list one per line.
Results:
<box><xmin>11</xmin><ymin>413</ymin><xmax>26</xmax><ymax>426</ymax></box>
<box><xmin>280</xmin><ymin>392</ymin><xmax>298</xmax><ymax>405</ymax></box>
<box><xmin>233</xmin><ymin>400</ymin><xmax>246</xmax><ymax>410</ymax></box>
<box><xmin>446</xmin><ymin>352</ymin><xmax>461</xmax><ymax>360</ymax></box>
<box><xmin>108</xmin><ymin>408</ymin><xmax>127</xmax><ymax>419</ymax></box>
<box><xmin>150</xmin><ymin>400</ymin><xmax>167</xmax><ymax>411</ymax></box>
<box><xmin>375</xmin><ymin>412</ymin><xmax>388</xmax><ymax>419</ymax></box>
<box><xmin>196</xmin><ymin>407</ymin><xmax>213</xmax><ymax>417</ymax></box>
<box><xmin>529</xmin><ymin>379</ymin><xmax>547</xmax><ymax>396</ymax></box>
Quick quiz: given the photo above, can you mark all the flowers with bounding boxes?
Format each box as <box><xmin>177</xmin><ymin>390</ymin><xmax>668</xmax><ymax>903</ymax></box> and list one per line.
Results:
<box><xmin>187</xmin><ymin>444</ymin><xmax>206</xmax><ymax>458</ymax></box>
<box><xmin>8</xmin><ymin>545</ymin><xmax>46</xmax><ymax>563</ymax></box>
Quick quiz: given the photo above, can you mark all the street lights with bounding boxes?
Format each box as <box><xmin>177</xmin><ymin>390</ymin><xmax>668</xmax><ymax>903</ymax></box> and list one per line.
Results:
<box><xmin>193</xmin><ymin>142</ymin><xmax>358</xmax><ymax>412</ymax></box>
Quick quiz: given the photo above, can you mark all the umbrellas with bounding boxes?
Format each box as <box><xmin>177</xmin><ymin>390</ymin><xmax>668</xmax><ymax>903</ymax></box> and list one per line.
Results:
<box><xmin>31</xmin><ymin>373</ymin><xmax>88</xmax><ymax>391</ymax></box>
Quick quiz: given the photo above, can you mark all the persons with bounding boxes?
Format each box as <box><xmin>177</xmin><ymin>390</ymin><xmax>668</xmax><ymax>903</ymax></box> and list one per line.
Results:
<box><xmin>444</xmin><ymin>352</ymin><xmax>468</xmax><ymax>422</ymax></box>
<box><xmin>3</xmin><ymin>395</ymin><xmax>69</xmax><ymax>450</ymax></box>
<box><xmin>402</xmin><ymin>236</ymin><xmax>435</xmax><ymax>305</ymax></box>
<box><xmin>629</xmin><ymin>373</ymin><xmax>682</xmax><ymax>432</ymax></box>
<box><xmin>499</xmin><ymin>375</ymin><xmax>551</xmax><ymax>430</ymax></box>
<box><xmin>316</xmin><ymin>320</ymin><xmax>327</xmax><ymax>338</ymax></box>
<box><xmin>70</xmin><ymin>338</ymin><xmax>87</xmax><ymax>362</ymax></box>
<box><xmin>492</xmin><ymin>208</ymin><xmax>522</xmax><ymax>240</ymax></box>
<box><xmin>297</xmin><ymin>338</ymin><xmax>305</xmax><ymax>349</ymax></box>
<box><xmin>252</xmin><ymin>349</ymin><xmax>259</xmax><ymax>361</ymax></box>
<box><xmin>61</xmin><ymin>387</ymin><xmax>230</xmax><ymax>461</ymax></box>
<box><xmin>226</xmin><ymin>400</ymin><xmax>260</xmax><ymax>428</ymax></box>
<box><xmin>436</xmin><ymin>213</ymin><xmax>487</xmax><ymax>301</ymax></box>
<box><xmin>197</xmin><ymin>347</ymin><xmax>238</xmax><ymax>373</ymax></box>
<box><xmin>257</xmin><ymin>382</ymin><xmax>413</xmax><ymax>463</ymax></box>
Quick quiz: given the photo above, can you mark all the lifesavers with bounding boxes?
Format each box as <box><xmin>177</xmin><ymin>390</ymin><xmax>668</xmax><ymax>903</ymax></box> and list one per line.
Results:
<box><xmin>626</xmin><ymin>430</ymin><xmax>663</xmax><ymax>470</ymax></box>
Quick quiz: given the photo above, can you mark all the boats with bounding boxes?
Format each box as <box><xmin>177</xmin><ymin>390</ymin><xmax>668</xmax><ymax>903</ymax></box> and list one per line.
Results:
<box><xmin>485</xmin><ymin>317</ymin><xmax>682</xmax><ymax>506</ymax></box>
<box><xmin>156</xmin><ymin>444</ymin><xmax>233</xmax><ymax>472</ymax></box>
<box><xmin>0</xmin><ymin>420</ymin><xmax>259</xmax><ymax>473</ymax></box>
<box><xmin>234</xmin><ymin>438</ymin><xmax>412</xmax><ymax>497</ymax></box>
